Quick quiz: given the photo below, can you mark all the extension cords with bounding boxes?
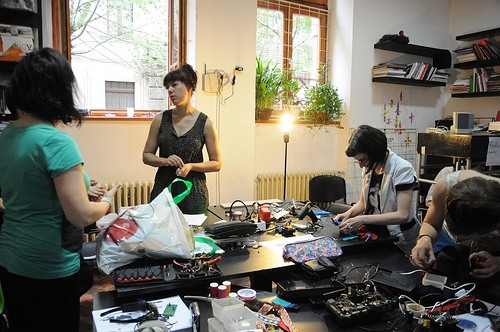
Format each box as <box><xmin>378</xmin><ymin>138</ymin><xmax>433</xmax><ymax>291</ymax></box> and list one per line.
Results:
<box><xmin>250</xmin><ymin>219</ymin><xmax>266</xmax><ymax>232</ymax></box>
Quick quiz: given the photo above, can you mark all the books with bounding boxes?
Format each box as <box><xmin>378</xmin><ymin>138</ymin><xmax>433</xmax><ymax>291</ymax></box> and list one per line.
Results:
<box><xmin>372</xmin><ymin>37</ymin><xmax>500</xmax><ymax>93</ymax></box>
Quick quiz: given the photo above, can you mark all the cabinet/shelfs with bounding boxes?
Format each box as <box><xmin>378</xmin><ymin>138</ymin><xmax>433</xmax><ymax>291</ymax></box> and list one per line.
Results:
<box><xmin>451</xmin><ymin>27</ymin><xmax>500</xmax><ymax>98</ymax></box>
<box><xmin>415</xmin><ymin>132</ymin><xmax>500</xmax><ymax>224</ymax></box>
<box><xmin>0</xmin><ymin>6</ymin><xmax>39</xmax><ymax>122</ymax></box>
<box><xmin>372</xmin><ymin>40</ymin><xmax>452</xmax><ymax>87</ymax></box>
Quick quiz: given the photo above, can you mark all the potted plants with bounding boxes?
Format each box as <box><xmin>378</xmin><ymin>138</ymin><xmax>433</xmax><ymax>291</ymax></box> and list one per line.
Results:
<box><xmin>255</xmin><ymin>52</ymin><xmax>302</xmax><ymax>122</ymax></box>
<box><xmin>303</xmin><ymin>63</ymin><xmax>345</xmax><ymax>133</ymax></box>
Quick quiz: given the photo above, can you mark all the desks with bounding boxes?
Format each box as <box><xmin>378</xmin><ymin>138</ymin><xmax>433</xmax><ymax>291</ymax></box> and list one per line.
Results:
<box><xmin>93</xmin><ymin>203</ymin><xmax>460</xmax><ymax>332</ymax></box>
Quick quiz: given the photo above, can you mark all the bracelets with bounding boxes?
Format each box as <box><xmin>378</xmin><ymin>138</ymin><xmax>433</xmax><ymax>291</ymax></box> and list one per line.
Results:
<box><xmin>100</xmin><ymin>197</ymin><xmax>113</xmax><ymax>206</ymax></box>
<box><xmin>417</xmin><ymin>233</ymin><xmax>433</xmax><ymax>240</ymax></box>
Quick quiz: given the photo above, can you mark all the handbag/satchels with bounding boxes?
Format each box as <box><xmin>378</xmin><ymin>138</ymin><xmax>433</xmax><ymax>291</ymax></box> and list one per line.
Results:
<box><xmin>93</xmin><ymin>178</ymin><xmax>226</xmax><ymax>273</ymax></box>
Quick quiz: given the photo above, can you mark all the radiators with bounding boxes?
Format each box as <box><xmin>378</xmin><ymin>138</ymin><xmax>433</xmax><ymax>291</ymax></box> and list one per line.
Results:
<box><xmin>83</xmin><ymin>181</ymin><xmax>155</xmax><ymax>243</ymax></box>
<box><xmin>254</xmin><ymin>171</ymin><xmax>344</xmax><ymax>202</ymax></box>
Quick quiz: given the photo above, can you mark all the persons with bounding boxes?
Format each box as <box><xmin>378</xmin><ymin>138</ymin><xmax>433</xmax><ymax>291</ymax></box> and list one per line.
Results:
<box><xmin>333</xmin><ymin>125</ymin><xmax>421</xmax><ymax>257</ymax></box>
<box><xmin>0</xmin><ymin>48</ymin><xmax>122</xmax><ymax>332</ymax></box>
<box><xmin>412</xmin><ymin>170</ymin><xmax>500</xmax><ymax>307</ymax></box>
<box><xmin>142</xmin><ymin>64</ymin><xmax>222</xmax><ymax>214</ymax></box>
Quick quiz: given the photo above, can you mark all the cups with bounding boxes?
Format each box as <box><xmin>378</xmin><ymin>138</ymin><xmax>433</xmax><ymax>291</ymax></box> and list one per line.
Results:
<box><xmin>406</xmin><ymin>303</ymin><xmax>425</xmax><ymax>318</ymax></box>
<box><xmin>209</xmin><ymin>280</ymin><xmax>232</xmax><ymax>299</ymax></box>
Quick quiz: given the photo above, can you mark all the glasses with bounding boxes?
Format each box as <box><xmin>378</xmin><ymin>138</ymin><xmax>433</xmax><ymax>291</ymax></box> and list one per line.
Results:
<box><xmin>353</xmin><ymin>155</ymin><xmax>370</xmax><ymax>166</ymax></box>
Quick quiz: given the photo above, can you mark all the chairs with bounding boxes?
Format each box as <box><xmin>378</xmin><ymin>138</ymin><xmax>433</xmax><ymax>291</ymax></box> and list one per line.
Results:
<box><xmin>309</xmin><ymin>175</ymin><xmax>347</xmax><ymax>210</ymax></box>
